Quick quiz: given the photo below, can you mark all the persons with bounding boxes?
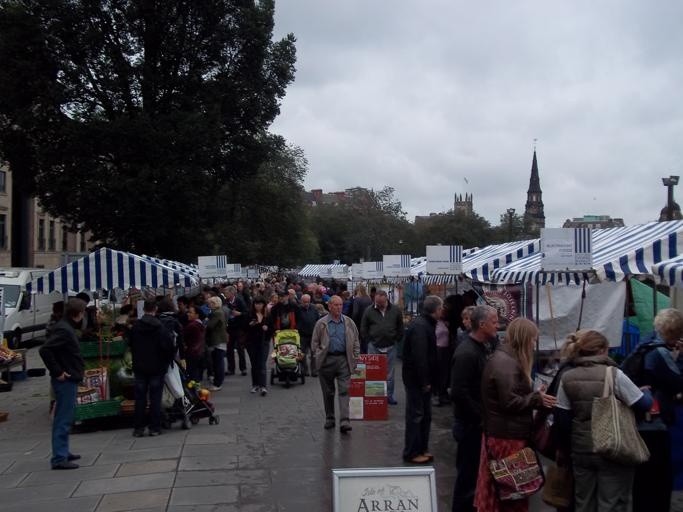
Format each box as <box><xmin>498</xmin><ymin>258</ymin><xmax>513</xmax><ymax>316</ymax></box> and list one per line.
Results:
<box><xmin>40</xmin><ymin>277</ymin><xmax>682</xmax><ymax>512</ymax></box>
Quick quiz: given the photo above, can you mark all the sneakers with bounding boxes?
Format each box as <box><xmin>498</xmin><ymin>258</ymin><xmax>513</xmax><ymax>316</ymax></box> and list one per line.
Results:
<box><xmin>325</xmin><ymin>423</ymin><xmax>335</xmax><ymax>428</ymax></box>
<box><xmin>341</xmin><ymin>425</ymin><xmax>352</xmax><ymax>432</ymax></box>
<box><xmin>250</xmin><ymin>386</ymin><xmax>267</xmax><ymax>395</ymax></box>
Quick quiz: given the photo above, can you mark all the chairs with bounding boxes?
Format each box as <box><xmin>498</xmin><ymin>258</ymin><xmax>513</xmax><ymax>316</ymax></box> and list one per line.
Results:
<box><xmin>0</xmin><ymin>340</ymin><xmax>33</xmax><ymax>382</ymax></box>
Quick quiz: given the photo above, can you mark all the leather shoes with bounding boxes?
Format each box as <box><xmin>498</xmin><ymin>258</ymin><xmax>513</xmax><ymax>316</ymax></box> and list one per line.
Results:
<box><xmin>403</xmin><ymin>453</ymin><xmax>434</xmax><ymax>463</ymax></box>
<box><xmin>52</xmin><ymin>453</ymin><xmax>80</xmax><ymax>469</ymax></box>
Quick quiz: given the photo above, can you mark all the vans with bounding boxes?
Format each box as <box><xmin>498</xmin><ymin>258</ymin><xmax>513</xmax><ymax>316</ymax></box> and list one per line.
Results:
<box><xmin>0</xmin><ymin>266</ymin><xmax>67</xmax><ymax>350</ymax></box>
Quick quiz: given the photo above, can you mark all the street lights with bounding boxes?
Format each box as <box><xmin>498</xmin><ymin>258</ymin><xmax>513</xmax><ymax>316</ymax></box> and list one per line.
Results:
<box><xmin>506</xmin><ymin>207</ymin><xmax>516</xmax><ymax>242</ymax></box>
<box><xmin>398</xmin><ymin>240</ymin><xmax>402</xmax><ymax>255</ymax></box>
<box><xmin>659</xmin><ymin>175</ymin><xmax>681</xmax><ymax>221</ymax></box>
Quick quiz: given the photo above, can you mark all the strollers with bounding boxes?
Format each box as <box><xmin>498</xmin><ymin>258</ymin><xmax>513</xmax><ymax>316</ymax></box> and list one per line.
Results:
<box><xmin>173</xmin><ymin>358</ymin><xmax>219</xmax><ymax>425</ymax></box>
<box><xmin>270</xmin><ymin>329</ymin><xmax>306</xmax><ymax>388</ymax></box>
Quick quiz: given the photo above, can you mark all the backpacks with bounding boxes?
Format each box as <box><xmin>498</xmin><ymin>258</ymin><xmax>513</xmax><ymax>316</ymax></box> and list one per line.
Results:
<box><xmin>620</xmin><ymin>342</ymin><xmax>672</xmax><ymax>384</ymax></box>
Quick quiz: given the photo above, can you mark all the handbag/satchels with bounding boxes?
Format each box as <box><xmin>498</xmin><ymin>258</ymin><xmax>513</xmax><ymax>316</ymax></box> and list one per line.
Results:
<box><xmin>489</xmin><ymin>447</ymin><xmax>545</xmax><ymax>501</ymax></box>
<box><xmin>591</xmin><ymin>366</ymin><xmax>650</xmax><ymax>465</ymax></box>
